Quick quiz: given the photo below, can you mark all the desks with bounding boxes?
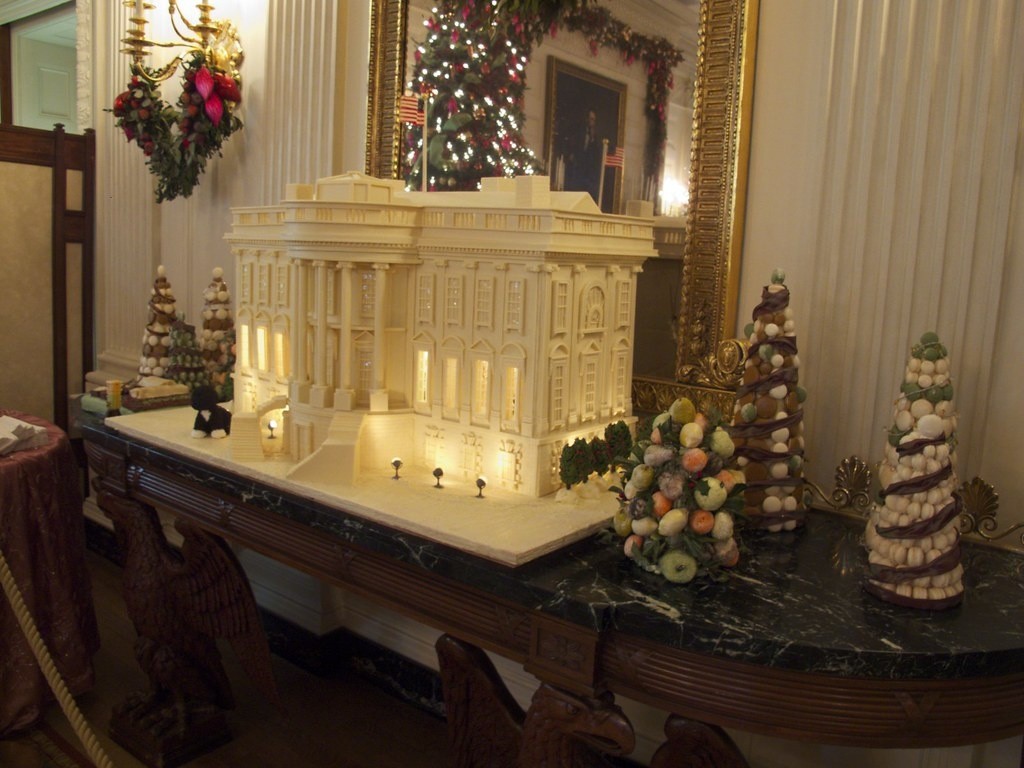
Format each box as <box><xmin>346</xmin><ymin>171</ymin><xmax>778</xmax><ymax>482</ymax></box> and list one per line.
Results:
<box><xmin>0</xmin><ymin>407</ymin><xmax>103</xmax><ymax>743</ymax></box>
<box><xmin>75</xmin><ymin>397</ymin><xmax>1024</xmax><ymax>768</ymax></box>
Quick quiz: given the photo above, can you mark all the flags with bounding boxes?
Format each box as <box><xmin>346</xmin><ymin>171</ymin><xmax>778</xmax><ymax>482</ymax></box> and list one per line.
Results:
<box><xmin>399</xmin><ymin>96</ymin><xmax>427</xmax><ymax>126</ymax></box>
<box><xmin>605</xmin><ymin>144</ymin><xmax>625</xmax><ymax>170</ymax></box>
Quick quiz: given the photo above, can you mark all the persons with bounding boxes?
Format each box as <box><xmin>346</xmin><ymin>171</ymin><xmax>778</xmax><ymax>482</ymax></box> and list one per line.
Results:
<box><xmin>570</xmin><ymin>104</ymin><xmax>606</xmax><ymax>207</ymax></box>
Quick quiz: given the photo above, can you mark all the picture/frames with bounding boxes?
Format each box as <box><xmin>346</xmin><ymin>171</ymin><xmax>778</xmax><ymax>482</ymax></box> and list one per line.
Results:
<box><xmin>544</xmin><ymin>53</ymin><xmax>628</xmax><ymax>216</ymax></box>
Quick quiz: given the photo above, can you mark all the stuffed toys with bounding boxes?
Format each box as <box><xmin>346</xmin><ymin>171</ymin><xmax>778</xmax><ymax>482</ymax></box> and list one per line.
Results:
<box><xmin>190</xmin><ymin>386</ymin><xmax>231</xmax><ymax>439</ymax></box>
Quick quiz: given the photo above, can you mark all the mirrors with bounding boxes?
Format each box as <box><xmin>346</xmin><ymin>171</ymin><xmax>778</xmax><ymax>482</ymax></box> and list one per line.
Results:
<box><xmin>363</xmin><ymin>0</ymin><xmax>759</xmax><ymax>428</ymax></box>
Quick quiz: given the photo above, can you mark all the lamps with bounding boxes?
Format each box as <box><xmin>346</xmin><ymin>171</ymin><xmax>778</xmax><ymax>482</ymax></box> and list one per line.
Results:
<box><xmin>267</xmin><ymin>420</ymin><xmax>488</xmax><ymax>501</ymax></box>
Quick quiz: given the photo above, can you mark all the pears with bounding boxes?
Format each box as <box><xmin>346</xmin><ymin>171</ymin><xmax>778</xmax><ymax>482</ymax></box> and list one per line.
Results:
<box><xmin>614</xmin><ymin>398</ymin><xmax>747</xmax><ymax>582</ymax></box>
